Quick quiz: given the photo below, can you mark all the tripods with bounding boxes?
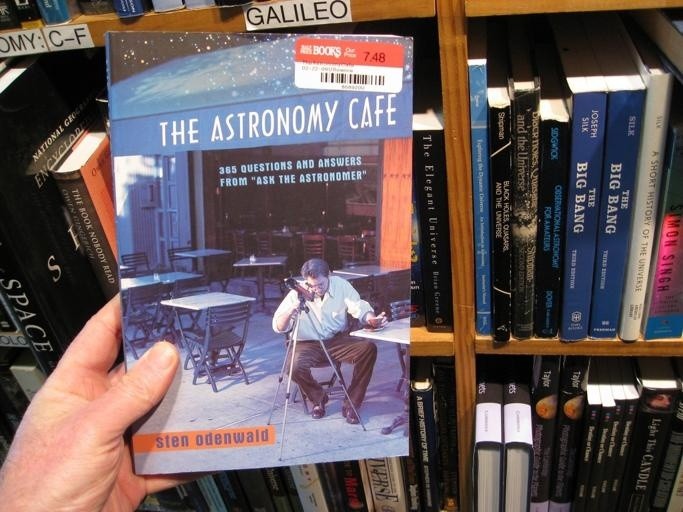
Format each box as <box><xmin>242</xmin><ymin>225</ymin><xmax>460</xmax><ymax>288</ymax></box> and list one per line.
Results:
<box><xmin>268</xmin><ymin>301</ymin><xmax>366</xmax><ymax>461</ymax></box>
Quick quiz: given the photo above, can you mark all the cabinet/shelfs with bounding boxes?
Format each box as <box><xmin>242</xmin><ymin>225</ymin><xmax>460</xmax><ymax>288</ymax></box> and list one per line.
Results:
<box><xmin>1</xmin><ymin>1</ymin><xmax>683</xmax><ymax>512</ymax></box>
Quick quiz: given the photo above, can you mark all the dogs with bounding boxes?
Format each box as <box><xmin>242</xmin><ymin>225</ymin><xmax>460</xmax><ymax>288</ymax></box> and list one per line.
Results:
<box><xmin>381</xmin><ymin>376</ymin><xmax>410</xmax><ymax>438</ymax></box>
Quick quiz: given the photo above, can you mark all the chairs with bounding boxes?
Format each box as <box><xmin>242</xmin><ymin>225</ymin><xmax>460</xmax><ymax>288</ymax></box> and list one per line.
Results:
<box><xmin>121</xmin><ymin>228</ymin><xmax>411</xmax><ymax>415</ymax></box>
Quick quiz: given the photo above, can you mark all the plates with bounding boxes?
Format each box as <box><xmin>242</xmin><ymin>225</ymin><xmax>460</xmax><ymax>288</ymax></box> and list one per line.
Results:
<box><xmin>362</xmin><ymin>327</ymin><xmax>384</xmax><ymax>332</ymax></box>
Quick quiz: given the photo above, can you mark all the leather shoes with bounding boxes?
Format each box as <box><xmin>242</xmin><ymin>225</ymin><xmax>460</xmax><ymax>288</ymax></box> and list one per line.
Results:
<box><xmin>312</xmin><ymin>393</ymin><xmax>329</xmax><ymax>418</ymax></box>
<box><xmin>342</xmin><ymin>406</ymin><xmax>359</xmax><ymax>423</ymax></box>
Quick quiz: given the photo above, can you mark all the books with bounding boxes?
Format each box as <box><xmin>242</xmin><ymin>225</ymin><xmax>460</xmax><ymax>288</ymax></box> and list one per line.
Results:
<box><xmin>2</xmin><ymin>0</ymin><xmax>234</xmax><ymax>30</ymax></box>
<box><xmin>135</xmin><ymin>455</ymin><xmax>411</xmax><ymax>511</ymax></box>
<box><xmin>105</xmin><ymin>28</ymin><xmax>412</xmax><ymax>475</ymax></box>
<box><xmin>413</xmin><ymin>356</ymin><xmax>683</xmax><ymax>511</ymax></box>
<box><xmin>1</xmin><ymin>56</ymin><xmax>125</xmax><ymax>461</ymax></box>
<box><xmin>412</xmin><ymin>10</ymin><xmax>683</xmax><ymax>344</ymax></box>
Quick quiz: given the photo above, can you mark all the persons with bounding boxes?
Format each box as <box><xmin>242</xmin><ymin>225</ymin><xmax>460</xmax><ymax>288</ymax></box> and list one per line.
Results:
<box><xmin>0</xmin><ymin>290</ymin><xmax>218</xmax><ymax>511</ymax></box>
<box><xmin>271</xmin><ymin>258</ymin><xmax>387</xmax><ymax>423</ymax></box>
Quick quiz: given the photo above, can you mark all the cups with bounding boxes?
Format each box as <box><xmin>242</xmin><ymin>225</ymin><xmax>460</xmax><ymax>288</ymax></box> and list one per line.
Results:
<box><xmin>367</xmin><ymin>317</ymin><xmax>384</xmax><ymax>329</ymax></box>
